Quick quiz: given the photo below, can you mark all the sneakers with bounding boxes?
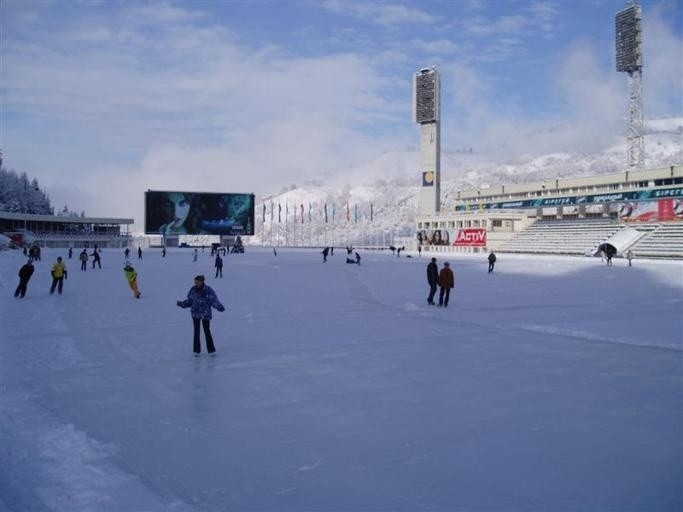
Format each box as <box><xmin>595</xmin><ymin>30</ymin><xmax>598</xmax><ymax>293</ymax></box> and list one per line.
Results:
<box><xmin>209</xmin><ymin>352</ymin><xmax>217</xmax><ymax>357</ymax></box>
<box><xmin>428</xmin><ymin>302</ymin><xmax>447</xmax><ymax>307</ymax></box>
<box><xmin>194</xmin><ymin>352</ymin><xmax>201</xmax><ymax>357</ymax></box>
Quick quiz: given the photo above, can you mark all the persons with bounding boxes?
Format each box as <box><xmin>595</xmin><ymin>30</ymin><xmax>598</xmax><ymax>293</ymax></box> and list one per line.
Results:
<box><xmin>396</xmin><ymin>247</ymin><xmax>402</xmax><ymax>257</ymax></box>
<box><xmin>388</xmin><ymin>245</ymin><xmax>396</xmax><ymax>256</ymax></box>
<box><xmin>416</xmin><ymin>230</ymin><xmax>428</xmax><ymax>245</ymax></box>
<box><xmin>598</xmin><ymin>247</ymin><xmax>604</xmax><ymax>262</ymax></box>
<box><xmin>349</xmin><ymin>244</ymin><xmax>355</xmax><ymax>253</ymax></box>
<box><xmin>155</xmin><ymin>191</ymin><xmax>206</xmax><ymax>234</ymax></box>
<box><xmin>22</xmin><ymin>238</ymin><xmax>245</xmax><ymax>271</ymax></box>
<box><xmin>487</xmin><ymin>250</ymin><xmax>496</xmax><ymax>274</ymax></box>
<box><xmin>273</xmin><ymin>247</ymin><xmax>276</xmax><ymax>256</ymax></box>
<box><xmin>437</xmin><ymin>261</ymin><xmax>454</xmax><ymax>308</ymax></box>
<box><xmin>417</xmin><ymin>246</ymin><xmax>420</xmax><ymax>257</ymax></box>
<box><xmin>425</xmin><ymin>256</ymin><xmax>438</xmax><ymax>304</ymax></box>
<box><xmin>442</xmin><ymin>231</ymin><xmax>450</xmax><ymax>244</ymax></box>
<box><xmin>214</xmin><ymin>253</ymin><xmax>223</xmax><ymax>279</ymax></box>
<box><xmin>405</xmin><ymin>255</ymin><xmax>412</xmax><ymax>258</ymax></box>
<box><xmin>345</xmin><ymin>256</ymin><xmax>355</xmax><ymax>264</ymax></box>
<box><xmin>354</xmin><ymin>251</ymin><xmax>360</xmax><ymax>266</ymax></box>
<box><xmin>48</xmin><ymin>256</ymin><xmax>68</xmax><ymax>297</ymax></box>
<box><xmin>121</xmin><ymin>260</ymin><xmax>141</xmax><ymax>299</ymax></box>
<box><xmin>330</xmin><ymin>246</ymin><xmax>333</xmax><ymax>256</ymax></box>
<box><xmin>345</xmin><ymin>244</ymin><xmax>351</xmax><ymax>255</ymax></box>
<box><xmin>319</xmin><ymin>246</ymin><xmax>329</xmax><ymax>263</ymax></box>
<box><xmin>625</xmin><ymin>249</ymin><xmax>632</xmax><ymax>266</ymax></box>
<box><xmin>13</xmin><ymin>258</ymin><xmax>34</xmax><ymax>299</ymax></box>
<box><xmin>605</xmin><ymin>248</ymin><xmax>612</xmax><ymax>267</ymax></box>
<box><xmin>175</xmin><ymin>274</ymin><xmax>226</xmax><ymax>356</ymax></box>
<box><xmin>429</xmin><ymin>229</ymin><xmax>444</xmax><ymax>244</ymax></box>
<box><xmin>401</xmin><ymin>246</ymin><xmax>404</xmax><ymax>250</ymax></box>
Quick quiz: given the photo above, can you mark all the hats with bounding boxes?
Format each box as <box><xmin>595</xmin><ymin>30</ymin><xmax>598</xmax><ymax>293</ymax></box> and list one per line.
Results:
<box><xmin>445</xmin><ymin>262</ymin><xmax>448</xmax><ymax>266</ymax></box>
<box><xmin>431</xmin><ymin>258</ymin><xmax>435</xmax><ymax>261</ymax></box>
<box><xmin>195</xmin><ymin>275</ymin><xmax>204</xmax><ymax>281</ymax></box>
<box><xmin>27</xmin><ymin>260</ymin><xmax>33</xmax><ymax>263</ymax></box>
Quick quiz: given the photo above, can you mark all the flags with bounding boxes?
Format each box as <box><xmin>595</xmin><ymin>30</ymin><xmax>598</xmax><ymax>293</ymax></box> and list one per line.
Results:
<box><xmin>260</xmin><ymin>198</ymin><xmax>373</xmax><ymax>225</ymax></box>
